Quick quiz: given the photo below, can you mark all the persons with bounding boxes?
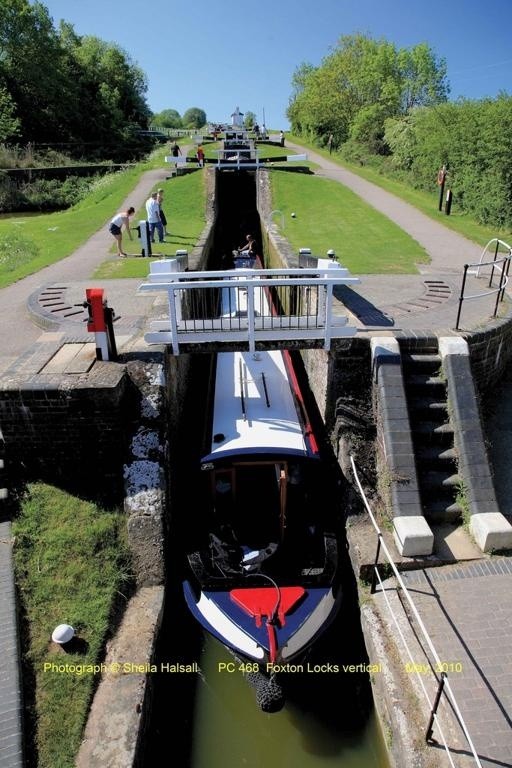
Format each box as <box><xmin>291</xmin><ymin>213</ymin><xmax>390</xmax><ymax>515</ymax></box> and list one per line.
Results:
<box><xmin>195</xmin><ymin>142</ymin><xmax>206</xmax><ymax>168</ymax></box>
<box><xmin>237</xmin><ymin>233</ymin><xmax>259</xmax><ymax>257</ymax></box>
<box><xmin>144</xmin><ymin>190</ymin><xmax>168</xmax><ymax>243</ymax></box>
<box><xmin>279</xmin><ymin>130</ymin><xmax>285</xmax><ymax>138</ymax></box>
<box><xmin>260</xmin><ymin>124</ymin><xmax>267</xmax><ymax>141</ymax></box>
<box><xmin>107</xmin><ymin>206</ymin><xmax>137</xmax><ymax>259</ymax></box>
<box><xmin>169</xmin><ymin>140</ymin><xmax>183</xmax><ymax>169</ymax></box>
<box><xmin>155</xmin><ymin>187</ymin><xmax>171</xmax><ymax>237</ymax></box>
<box><xmin>252</xmin><ymin>122</ymin><xmax>261</xmax><ymax>140</ymax></box>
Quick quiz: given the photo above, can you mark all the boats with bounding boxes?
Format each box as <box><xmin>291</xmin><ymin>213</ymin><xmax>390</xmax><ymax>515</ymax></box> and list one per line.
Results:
<box><xmin>181</xmin><ymin>252</ymin><xmax>344</xmax><ymax>713</ymax></box>
